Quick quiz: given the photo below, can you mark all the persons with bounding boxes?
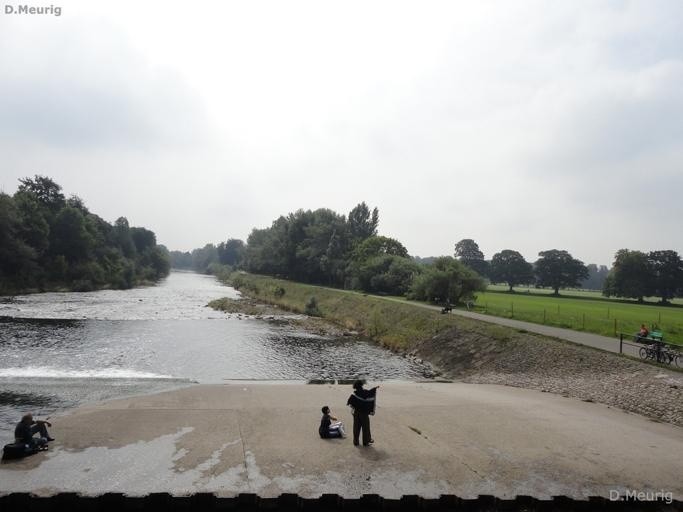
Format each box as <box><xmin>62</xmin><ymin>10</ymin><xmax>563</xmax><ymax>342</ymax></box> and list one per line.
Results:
<box><xmin>15</xmin><ymin>415</ymin><xmax>55</xmax><ymax>445</ymax></box>
<box><xmin>347</xmin><ymin>380</ymin><xmax>379</xmax><ymax>446</ymax></box>
<box><xmin>319</xmin><ymin>406</ymin><xmax>347</xmax><ymax>438</ymax></box>
<box><xmin>633</xmin><ymin>324</ymin><xmax>648</xmax><ymax>342</ymax></box>
<box><xmin>647</xmin><ymin>337</ymin><xmax>664</xmax><ymax>349</ymax></box>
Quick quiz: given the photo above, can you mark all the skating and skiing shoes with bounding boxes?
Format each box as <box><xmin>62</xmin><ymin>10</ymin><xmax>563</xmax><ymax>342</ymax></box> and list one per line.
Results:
<box><xmin>42</xmin><ymin>436</ymin><xmax>55</xmax><ymax>442</ymax></box>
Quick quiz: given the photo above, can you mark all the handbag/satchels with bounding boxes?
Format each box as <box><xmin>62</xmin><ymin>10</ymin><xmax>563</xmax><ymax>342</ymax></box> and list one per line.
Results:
<box><xmin>1</xmin><ymin>439</ymin><xmax>40</xmax><ymax>464</ymax></box>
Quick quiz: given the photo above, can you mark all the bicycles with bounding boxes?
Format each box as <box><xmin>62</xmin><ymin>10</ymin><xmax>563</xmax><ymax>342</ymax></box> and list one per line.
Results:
<box><xmin>638</xmin><ymin>340</ymin><xmax>683</xmax><ymax>369</ymax></box>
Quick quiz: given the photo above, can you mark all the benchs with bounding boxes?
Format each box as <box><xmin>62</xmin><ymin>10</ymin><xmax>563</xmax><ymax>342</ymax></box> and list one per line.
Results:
<box><xmin>635</xmin><ymin>331</ymin><xmax>663</xmax><ymax>346</ymax></box>
